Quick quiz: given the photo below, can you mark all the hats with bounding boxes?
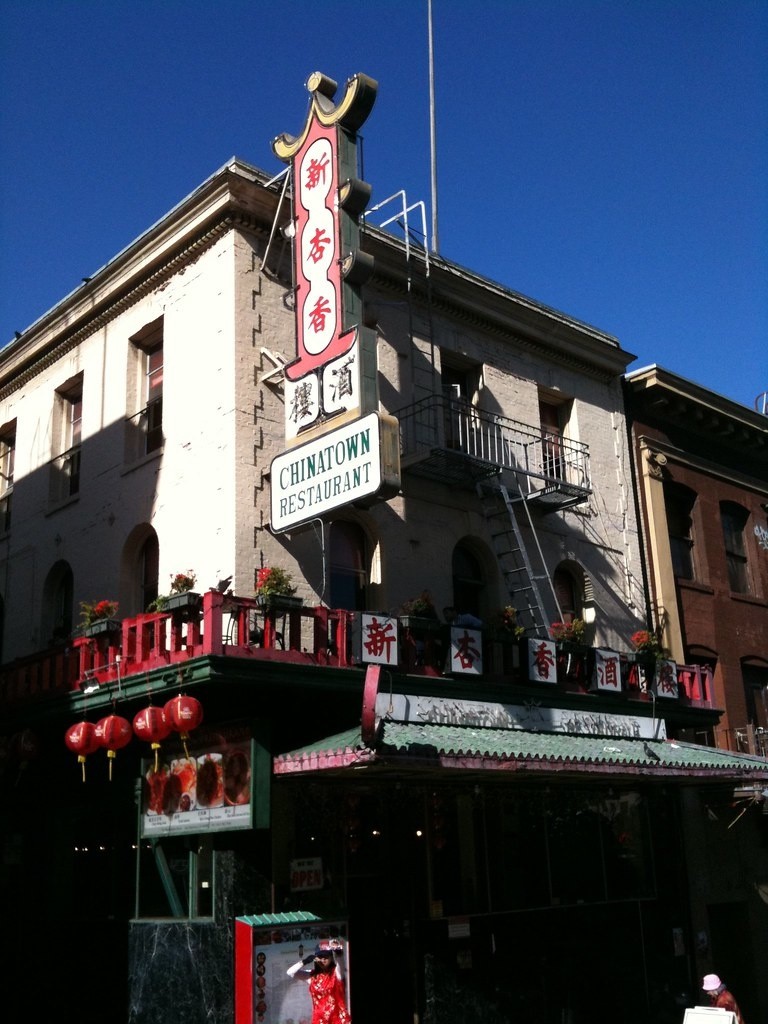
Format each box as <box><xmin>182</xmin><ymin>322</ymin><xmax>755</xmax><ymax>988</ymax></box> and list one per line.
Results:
<box><xmin>314</xmin><ymin>939</ymin><xmax>332</xmax><ymax>956</ymax></box>
<box><xmin>702</xmin><ymin>973</ymin><xmax>722</xmax><ymax>991</ymax></box>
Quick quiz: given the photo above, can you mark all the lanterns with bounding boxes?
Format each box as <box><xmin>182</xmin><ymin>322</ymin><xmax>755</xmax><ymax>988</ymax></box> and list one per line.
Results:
<box><xmin>161</xmin><ymin>697</ymin><xmax>203</xmax><ymax>739</ymax></box>
<box><xmin>95</xmin><ymin>716</ymin><xmax>133</xmax><ymax>757</ymax></box>
<box><xmin>134</xmin><ymin>707</ymin><xmax>171</xmax><ymax>748</ymax></box>
<box><xmin>65</xmin><ymin>722</ymin><xmax>98</xmax><ymax>761</ymax></box>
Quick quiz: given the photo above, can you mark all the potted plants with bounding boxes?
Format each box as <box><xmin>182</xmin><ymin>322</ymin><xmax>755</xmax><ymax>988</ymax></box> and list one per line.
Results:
<box><xmin>398</xmin><ymin>599</ymin><xmax>440</xmax><ymax>632</ymax></box>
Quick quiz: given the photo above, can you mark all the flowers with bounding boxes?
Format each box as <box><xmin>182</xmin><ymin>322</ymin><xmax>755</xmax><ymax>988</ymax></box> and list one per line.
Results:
<box><xmin>171</xmin><ymin>574</ymin><xmax>195</xmax><ymax>591</ymax></box>
<box><xmin>78</xmin><ymin>596</ymin><xmax>120</xmax><ymax>625</ymax></box>
<box><xmin>547</xmin><ymin>617</ymin><xmax>586</xmax><ymax>641</ymax></box>
<box><xmin>501</xmin><ymin>606</ymin><xmax>525</xmax><ymax>639</ymax></box>
<box><xmin>631</xmin><ymin>629</ymin><xmax>658</xmax><ymax>655</ymax></box>
<box><xmin>254</xmin><ymin>567</ymin><xmax>299</xmax><ymax>596</ymax></box>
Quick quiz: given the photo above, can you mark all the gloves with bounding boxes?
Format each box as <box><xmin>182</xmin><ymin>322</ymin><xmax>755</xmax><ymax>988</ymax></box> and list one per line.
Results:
<box><xmin>329</xmin><ymin>946</ymin><xmax>343</xmax><ymax>956</ymax></box>
<box><xmin>302</xmin><ymin>954</ymin><xmax>317</xmax><ymax>965</ymax></box>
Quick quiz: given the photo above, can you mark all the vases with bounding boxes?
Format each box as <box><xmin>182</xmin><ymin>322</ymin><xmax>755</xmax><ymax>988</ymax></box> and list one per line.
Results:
<box><xmin>558</xmin><ymin>639</ymin><xmax>587</xmax><ymax>654</ymax></box>
<box><xmin>627</xmin><ymin>652</ymin><xmax>653</xmax><ymax>663</ymax></box>
<box><xmin>161</xmin><ymin>592</ymin><xmax>201</xmax><ymax>611</ymax></box>
<box><xmin>255</xmin><ymin>593</ymin><xmax>303</xmax><ymax>610</ymax></box>
<box><xmin>83</xmin><ymin>618</ymin><xmax>121</xmax><ymax>638</ymax></box>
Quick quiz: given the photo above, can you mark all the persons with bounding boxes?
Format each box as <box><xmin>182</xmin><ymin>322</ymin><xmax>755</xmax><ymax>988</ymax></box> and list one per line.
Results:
<box><xmin>286</xmin><ymin>939</ymin><xmax>351</xmax><ymax>1024</ymax></box>
<box><xmin>702</xmin><ymin>974</ymin><xmax>745</xmax><ymax>1024</ymax></box>
<box><xmin>443</xmin><ymin>606</ymin><xmax>482</xmax><ymax>628</ymax></box>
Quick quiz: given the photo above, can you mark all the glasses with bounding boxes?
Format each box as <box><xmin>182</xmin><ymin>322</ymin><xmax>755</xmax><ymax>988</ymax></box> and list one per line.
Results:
<box><xmin>317</xmin><ymin>954</ymin><xmax>329</xmax><ymax>959</ymax></box>
